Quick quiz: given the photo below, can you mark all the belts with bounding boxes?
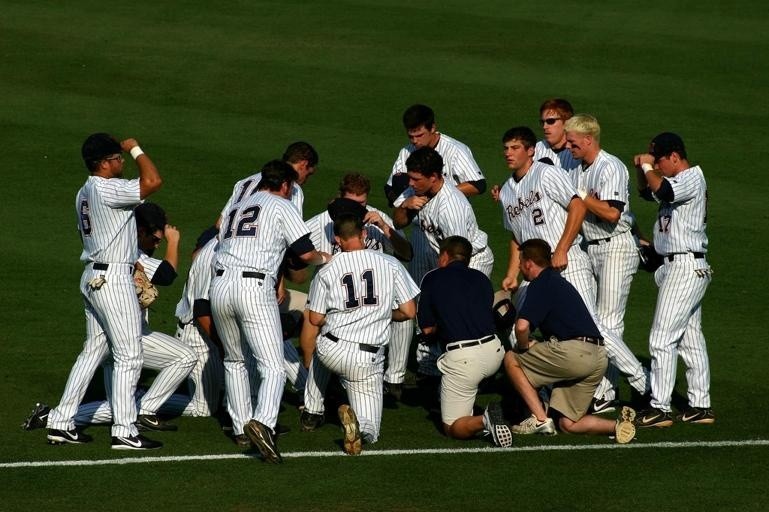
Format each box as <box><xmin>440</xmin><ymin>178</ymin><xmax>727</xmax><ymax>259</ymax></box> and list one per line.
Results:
<box><xmin>324</xmin><ymin>332</ymin><xmax>380</xmax><ymax>355</ymax></box>
<box><xmin>587</xmin><ymin>236</ymin><xmax>610</xmax><ymax>245</ymax></box>
<box><xmin>92</xmin><ymin>264</ymin><xmax>134</xmax><ymax>275</ymax></box>
<box><xmin>569</xmin><ymin>336</ymin><xmax>606</xmax><ymax>348</ymax></box>
<box><xmin>444</xmin><ymin>335</ymin><xmax>496</xmax><ymax>350</ymax></box>
<box><xmin>178</xmin><ymin>320</ymin><xmax>185</xmax><ymax>328</ymax></box>
<box><xmin>214</xmin><ymin>270</ymin><xmax>266</xmax><ymax>279</ymax></box>
<box><xmin>657</xmin><ymin>251</ymin><xmax>706</xmax><ymax>266</ymax></box>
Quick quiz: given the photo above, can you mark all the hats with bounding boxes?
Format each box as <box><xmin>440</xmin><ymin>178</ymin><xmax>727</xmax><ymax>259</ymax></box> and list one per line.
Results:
<box><xmin>262</xmin><ymin>161</ymin><xmax>299</xmax><ymax>183</ymax></box>
<box><xmin>327</xmin><ymin>197</ymin><xmax>369</xmax><ymax>225</ymax></box>
<box><xmin>649</xmin><ymin>133</ymin><xmax>683</xmax><ymax>161</ymax></box>
<box><xmin>81</xmin><ymin>131</ymin><xmax>127</xmax><ymax>160</ymax></box>
<box><xmin>139</xmin><ymin>203</ymin><xmax>168</xmax><ymax>231</ymax></box>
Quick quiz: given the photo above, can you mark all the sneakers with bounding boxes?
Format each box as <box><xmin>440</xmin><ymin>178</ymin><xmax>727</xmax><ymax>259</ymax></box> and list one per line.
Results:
<box><xmin>234</xmin><ymin>383</ymin><xmax>327</xmax><ymax>464</ymax></box>
<box><xmin>110</xmin><ymin>432</ymin><xmax>166</xmax><ymax>452</ymax></box>
<box><xmin>21</xmin><ymin>403</ymin><xmax>51</xmax><ymax>430</ymax></box>
<box><xmin>46</xmin><ymin>427</ymin><xmax>94</xmax><ymax>446</ymax></box>
<box><xmin>134</xmin><ymin>415</ymin><xmax>180</xmax><ymax>431</ymax></box>
<box><xmin>509</xmin><ymin>413</ymin><xmax>558</xmax><ymax>437</ymax></box>
<box><xmin>587</xmin><ymin>396</ymin><xmax>620</xmax><ymax>415</ymax></box>
<box><xmin>676</xmin><ymin>406</ymin><xmax>718</xmax><ymax>424</ymax></box>
<box><xmin>633</xmin><ymin>408</ymin><xmax>673</xmax><ymax>429</ymax></box>
<box><xmin>481</xmin><ymin>400</ymin><xmax>513</xmax><ymax>449</ymax></box>
<box><xmin>339</xmin><ymin>403</ymin><xmax>362</xmax><ymax>456</ymax></box>
<box><xmin>614</xmin><ymin>404</ymin><xmax>638</xmax><ymax>445</ymax></box>
<box><xmin>384</xmin><ymin>383</ymin><xmax>404</xmax><ymax>409</ymax></box>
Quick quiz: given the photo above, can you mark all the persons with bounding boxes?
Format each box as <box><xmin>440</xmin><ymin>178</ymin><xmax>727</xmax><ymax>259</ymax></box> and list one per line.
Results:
<box><xmin>21</xmin><ymin>200</ymin><xmax>199</xmax><ymax>432</ymax></box>
<box><xmin>176</xmin><ymin>98</ymin><xmax>652</xmax><ymax>445</ymax></box>
<box><xmin>44</xmin><ymin>133</ymin><xmax>164</xmax><ymax>451</ymax></box>
<box><xmin>634</xmin><ymin>132</ymin><xmax>714</xmax><ymax>427</ymax></box>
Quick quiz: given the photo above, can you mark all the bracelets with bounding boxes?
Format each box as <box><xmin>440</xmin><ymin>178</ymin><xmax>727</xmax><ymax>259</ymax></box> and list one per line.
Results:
<box><xmin>130</xmin><ymin>145</ymin><xmax>144</xmax><ymax>161</ymax></box>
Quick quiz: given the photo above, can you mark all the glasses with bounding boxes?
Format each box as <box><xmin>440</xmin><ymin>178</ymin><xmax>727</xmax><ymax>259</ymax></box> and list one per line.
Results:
<box><xmin>107</xmin><ymin>154</ymin><xmax>123</xmax><ymax>163</ymax></box>
<box><xmin>540</xmin><ymin>117</ymin><xmax>565</xmax><ymax>126</ymax></box>
